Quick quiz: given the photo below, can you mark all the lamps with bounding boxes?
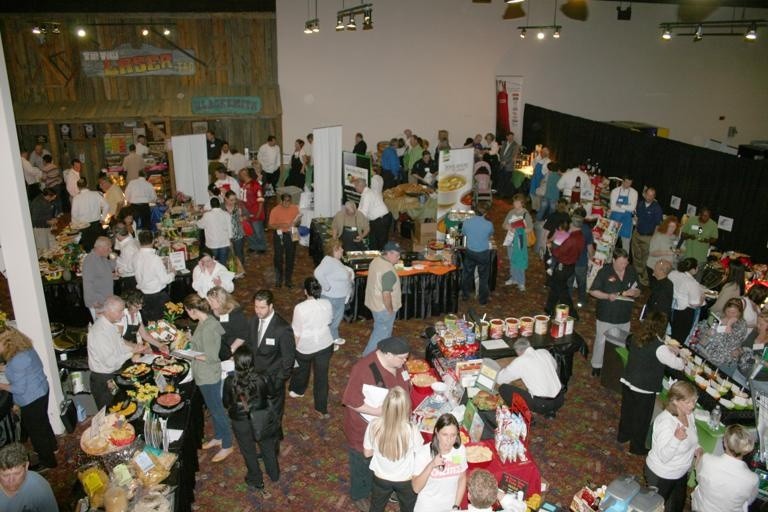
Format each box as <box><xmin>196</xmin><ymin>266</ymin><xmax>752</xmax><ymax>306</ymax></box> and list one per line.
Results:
<box><xmin>304</xmin><ymin>0</ymin><xmax>320</xmax><ymax>33</ymax></box>
<box><xmin>76</xmin><ymin>23</ymin><xmax>177</xmax><ymax>37</ymax></box>
<box><xmin>502</xmin><ymin>0</ymin><xmax>588</xmax><ymax>40</ymax></box>
<box><xmin>25</xmin><ymin>21</ymin><xmax>61</xmax><ymax>33</ymax></box>
<box><xmin>658</xmin><ymin>20</ymin><xmax>767</xmax><ymax>41</ymax></box>
<box><xmin>335</xmin><ymin>0</ymin><xmax>374</xmax><ymax>31</ymax></box>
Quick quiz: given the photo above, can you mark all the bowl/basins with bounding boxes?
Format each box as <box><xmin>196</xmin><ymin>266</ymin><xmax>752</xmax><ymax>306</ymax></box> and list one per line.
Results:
<box><xmin>438</xmin><ymin>173</ymin><xmax>468</xmax><ymax>206</ymax></box>
<box><xmin>430</xmin><ymin>381</ymin><xmax>448</xmax><ymax>395</ymax></box>
<box><xmin>463</xmin><ymin>445</ymin><xmax>494</xmax><ymax>463</ymax></box>
<box><xmin>47</xmin><ymin>321</ymin><xmax>65</xmax><ymax>338</ymax></box>
<box><xmin>666</xmin><ymin>335</ymin><xmax>753</xmax><ymax>411</ymax></box>
<box><xmin>401</xmin><ymin>262</ymin><xmax>426</xmax><ymax>274</ymax></box>
<box><xmin>85</xmin><ymin>434</ymin><xmax>109</xmax><ymax>454</ymax></box>
<box><xmin>104</xmin><ymin>356</ymin><xmax>190</xmax><ymax>420</ymax></box>
<box><xmin>108</xmin><ymin>427</ymin><xmax>134</xmax><ymax>446</ymax></box>
<box><xmin>405</xmin><ymin>358</ymin><xmax>437</xmax><ymax>393</ymax></box>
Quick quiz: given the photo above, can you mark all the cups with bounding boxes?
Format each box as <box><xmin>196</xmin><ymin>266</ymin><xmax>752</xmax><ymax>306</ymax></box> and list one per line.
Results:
<box><xmin>477</xmin><ymin>314</ymin><xmax>550</xmax><ymax>339</ymax></box>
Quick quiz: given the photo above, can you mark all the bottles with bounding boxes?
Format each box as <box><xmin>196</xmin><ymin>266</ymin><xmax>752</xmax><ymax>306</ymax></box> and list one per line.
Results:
<box><xmin>708</xmin><ymin>405</ymin><xmax>721</xmax><ymax>428</ymax></box>
<box><xmin>745</xmin><ymin>447</ymin><xmax>766</xmax><ymax>471</ymax></box>
<box><xmin>434</xmin><ymin>312</ymin><xmax>476</xmax><ymax>348</ymax></box>
<box><xmin>585</xmin><ymin>479</ymin><xmax>607</xmax><ymax>509</ymax></box>
<box><xmin>449</xmin><ymin>210</ymin><xmax>476</xmax><ymax>222</ymax></box>
<box><xmin>518</xmin><ymin>490</ymin><xmax>524</xmax><ymax>503</ymax></box>
<box><xmin>443</xmin><ymin>226</ymin><xmax>461</xmax><ymax>265</ymax></box>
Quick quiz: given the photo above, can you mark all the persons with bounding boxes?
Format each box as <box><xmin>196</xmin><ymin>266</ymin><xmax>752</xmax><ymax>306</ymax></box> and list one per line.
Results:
<box><xmin>291</xmin><ymin>276</ymin><xmax>336</xmax><ymax>418</ymax></box>
<box><xmin>314</xmin><ymin>129</ymin><xmax>660</xmax><ymax>358</ymax></box>
<box><xmin>22</xmin><ymin>133</ymin><xmax>315</xmax><ymax>323</ymax></box>
<box><xmin>341</xmin><ymin>337</ymin><xmax>498</xmax><ymax>511</ymax></box>
<box><xmin>185</xmin><ymin>288</ymin><xmax>297</xmax><ymax>489</ymax></box>
<box><xmin>589</xmin><ymin>209</ymin><xmax>768</xmax><ymax>512</ymax></box>
<box><xmin>89</xmin><ymin>296</ymin><xmax>169</xmax><ymax>418</ymax></box>
<box><xmin>1</xmin><ymin>325</ymin><xmax>59</xmax><ymax>512</ymax></box>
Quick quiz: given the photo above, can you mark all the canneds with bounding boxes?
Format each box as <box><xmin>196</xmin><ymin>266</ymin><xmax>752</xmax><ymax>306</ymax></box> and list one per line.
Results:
<box><xmin>435</xmin><ymin>302</ymin><xmax>575</xmax><ymax>345</ymax></box>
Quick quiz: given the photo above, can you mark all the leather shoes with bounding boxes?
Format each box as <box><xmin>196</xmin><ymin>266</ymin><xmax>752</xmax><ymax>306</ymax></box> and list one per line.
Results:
<box><xmin>202</xmin><ymin>439</ymin><xmax>222</xmax><ymax>449</ymax></box>
<box><xmin>211</xmin><ymin>447</ymin><xmax>232</xmax><ymax>462</ymax></box>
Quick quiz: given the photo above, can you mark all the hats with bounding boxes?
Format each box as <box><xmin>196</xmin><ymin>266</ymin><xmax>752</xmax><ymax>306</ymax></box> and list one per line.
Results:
<box><xmin>384</xmin><ymin>241</ymin><xmax>401</xmax><ymax>251</ymax></box>
<box><xmin>377</xmin><ymin>336</ymin><xmax>410</xmax><ymax>354</ymax></box>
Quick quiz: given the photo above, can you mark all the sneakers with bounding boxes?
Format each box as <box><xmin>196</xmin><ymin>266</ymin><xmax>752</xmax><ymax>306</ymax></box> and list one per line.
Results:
<box><xmin>289</xmin><ymin>391</ymin><xmax>304</xmax><ymax>399</ymax></box>
<box><xmin>529</xmin><ymin>416</ymin><xmax>536</xmax><ymax>426</ymax></box>
<box><xmin>33</xmin><ymin>447</ymin><xmax>59</xmax><ymax>456</ymax></box>
<box><xmin>357</xmin><ymin>497</ymin><xmax>371</xmax><ymax>511</ymax></box>
<box><xmin>334</xmin><ymin>345</ymin><xmax>339</xmax><ymax>352</ymax></box>
<box><xmin>33</xmin><ymin>461</ymin><xmax>55</xmax><ymax>473</ymax></box>
<box><xmin>591</xmin><ymin>368</ymin><xmax>600</xmax><ymax>377</ymax></box>
<box><xmin>245</xmin><ymin>476</ymin><xmax>265</xmax><ymax>488</ymax></box>
<box><xmin>276</xmin><ymin>281</ymin><xmax>282</xmax><ymax>287</ymax></box>
<box><xmin>334</xmin><ymin>338</ymin><xmax>346</xmax><ymax>345</ymax></box>
<box><xmin>520</xmin><ymin>286</ymin><xmax>525</xmax><ymax>292</ymax></box>
<box><xmin>543</xmin><ymin>410</ymin><xmax>555</xmax><ymax>418</ymax></box>
<box><xmin>505</xmin><ymin>279</ymin><xmax>514</xmax><ymax>285</ymax></box>
<box><xmin>285</xmin><ymin>280</ymin><xmax>294</xmax><ymax>288</ymax></box>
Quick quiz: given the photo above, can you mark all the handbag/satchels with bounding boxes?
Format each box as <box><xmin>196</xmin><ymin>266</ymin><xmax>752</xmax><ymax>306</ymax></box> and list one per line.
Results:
<box><xmin>526</xmin><ymin>230</ymin><xmax>536</xmax><ymax>247</ymax></box>
<box><xmin>250</xmin><ymin>405</ymin><xmax>282</xmax><ymax>442</ymax></box>
<box><xmin>59</xmin><ymin>399</ymin><xmax>79</xmax><ymax>434</ymax></box>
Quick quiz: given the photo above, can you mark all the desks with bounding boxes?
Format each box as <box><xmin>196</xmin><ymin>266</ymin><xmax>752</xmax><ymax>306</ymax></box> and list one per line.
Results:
<box><xmin>0</xmin><ymin>165</ymin><xmax>215</xmax><ymax>512</ymax></box>
<box><xmin>377</xmin><ymin>321</ymin><xmax>595</xmax><ymax>511</ymax></box>
<box><xmin>305</xmin><ymin>209</ymin><xmax>463</xmax><ymax>322</ymax></box>
<box><xmin>639</xmin><ymin>246</ymin><xmax>767</xmax><ymax>511</ymax></box>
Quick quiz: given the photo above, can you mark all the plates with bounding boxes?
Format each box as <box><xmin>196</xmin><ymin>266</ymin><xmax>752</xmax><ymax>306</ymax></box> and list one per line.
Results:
<box><xmin>425</xmin><ymin>252</ymin><xmax>444</xmax><ymax>262</ymax></box>
<box><xmin>131</xmin><ymin>481</ymin><xmax>170</xmax><ymax>511</ymax></box>
<box><xmin>429</xmin><ymin>241</ymin><xmax>447</xmax><ymax>251</ymax></box>
<box><xmin>141</xmin><ymin>412</ymin><xmax>170</xmax><ymax>450</ymax></box>
<box><xmin>704</xmin><ymin>288</ymin><xmax>720</xmax><ymax>300</ymax></box>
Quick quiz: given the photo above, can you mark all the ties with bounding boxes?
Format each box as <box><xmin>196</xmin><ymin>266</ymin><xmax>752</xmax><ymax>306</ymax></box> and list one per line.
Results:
<box><xmin>258</xmin><ymin>320</ymin><xmax>266</xmax><ymax>346</ymax></box>
